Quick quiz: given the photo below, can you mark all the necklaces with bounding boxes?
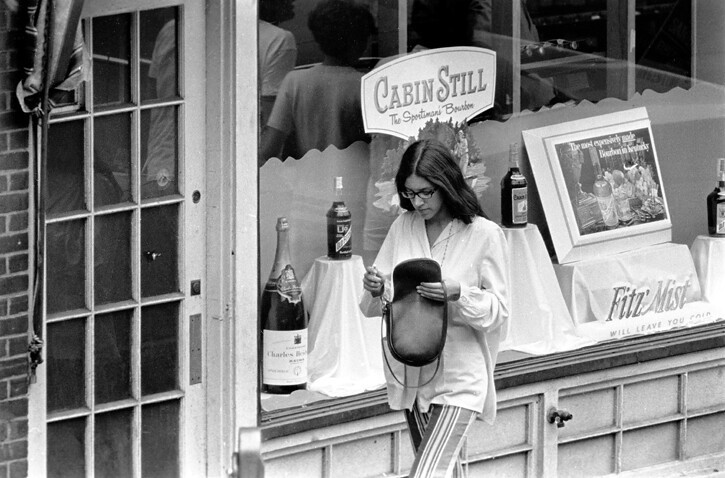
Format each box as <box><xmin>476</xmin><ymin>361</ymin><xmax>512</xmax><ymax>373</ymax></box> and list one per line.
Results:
<box><xmin>413</xmin><ymin>213</ymin><xmax>455</xmax><ymax>268</ymax></box>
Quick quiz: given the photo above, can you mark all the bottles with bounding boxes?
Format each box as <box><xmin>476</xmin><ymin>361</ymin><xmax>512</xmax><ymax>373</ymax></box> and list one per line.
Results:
<box><xmin>261</xmin><ymin>216</ymin><xmax>309</xmax><ymax>394</ymax></box>
<box><xmin>520</xmin><ymin>39</ymin><xmax>580</xmax><ymax>61</ymax></box>
<box><xmin>327</xmin><ymin>175</ymin><xmax>352</xmax><ymax>261</ymax></box>
<box><xmin>500</xmin><ymin>141</ymin><xmax>529</xmax><ymax>229</ymax></box>
<box><xmin>706</xmin><ymin>159</ymin><xmax>725</xmax><ymax>237</ymax></box>
<box><xmin>591</xmin><ymin>162</ymin><xmax>619</xmax><ymax>227</ymax></box>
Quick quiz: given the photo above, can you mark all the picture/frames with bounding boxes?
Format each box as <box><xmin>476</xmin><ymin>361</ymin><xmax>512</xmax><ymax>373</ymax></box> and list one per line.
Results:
<box><xmin>522</xmin><ymin>106</ymin><xmax>671</xmax><ymax>266</ymax></box>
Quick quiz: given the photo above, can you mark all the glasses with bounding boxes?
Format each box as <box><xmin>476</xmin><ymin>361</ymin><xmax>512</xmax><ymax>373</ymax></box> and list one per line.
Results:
<box><xmin>400</xmin><ymin>186</ymin><xmax>438</xmax><ymax>199</ymax></box>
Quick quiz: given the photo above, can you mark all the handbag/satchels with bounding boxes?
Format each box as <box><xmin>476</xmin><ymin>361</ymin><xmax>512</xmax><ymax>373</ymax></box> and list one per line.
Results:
<box><xmin>387</xmin><ymin>257</ymin><xmax>448</xmax><ymax>367</ymax></box>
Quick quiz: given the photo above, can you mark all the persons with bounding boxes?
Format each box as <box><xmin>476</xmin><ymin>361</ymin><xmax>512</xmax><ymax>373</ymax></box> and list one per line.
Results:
<box><xmin>257</xmin><ymin>0</ymin><xmax>607</xmax><ymax>170</ymax></box>
<box><xmin>31</xmin><ymin>18</ymin><xmax>178</xmax><ymax>478</ymax></box>
<box><xmin>359</xmin><ymin>139</ymin><xmax>512</xmax><ymax>478</ymax></box>
